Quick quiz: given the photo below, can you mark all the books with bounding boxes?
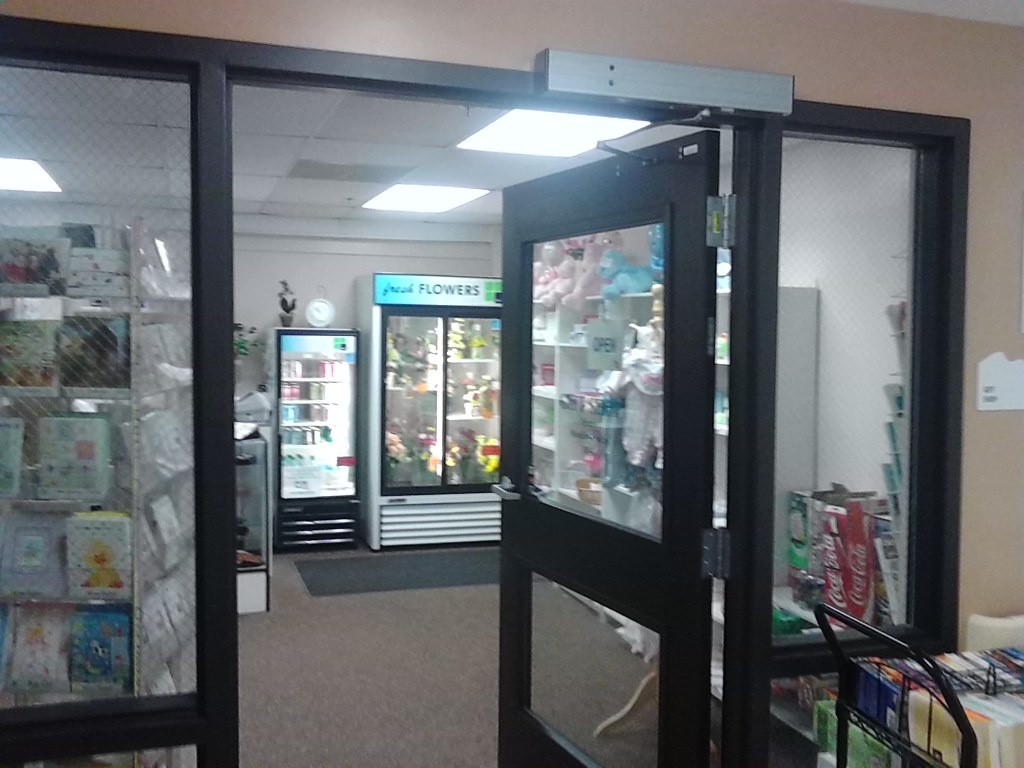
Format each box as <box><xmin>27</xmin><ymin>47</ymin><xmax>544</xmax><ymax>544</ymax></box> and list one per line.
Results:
<box><xmin>814</xmin><ymin>648</ymin><xmax>1024</xmax><ymax>768</ymax></box>
<box><xmin>0</xmin><ymin>224</ymin><xmax>131</xmax><ymax>705</ymax></box>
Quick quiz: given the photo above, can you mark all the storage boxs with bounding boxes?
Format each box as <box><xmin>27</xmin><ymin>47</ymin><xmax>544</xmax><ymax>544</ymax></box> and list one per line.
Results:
<box><xmin>787</xmin><ymin>483</ymin><xmax>894</xmax><ymax>631</ymax></box>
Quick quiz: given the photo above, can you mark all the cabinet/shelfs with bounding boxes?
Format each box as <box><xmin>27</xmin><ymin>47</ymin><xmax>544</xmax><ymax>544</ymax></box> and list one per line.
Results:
<box><xmin>530</xmin><ymin>285</ymin><xmax>817</xmax><ymax>701</ymax></box>
<box><xmin>0</xmin><ymin>221</ymin><xmax>195</xmax><ymax>768</ymax></box>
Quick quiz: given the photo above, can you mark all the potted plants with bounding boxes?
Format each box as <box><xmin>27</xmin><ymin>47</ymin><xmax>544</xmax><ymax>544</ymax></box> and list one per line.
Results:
<box><xmin>278</xmin><ymin>281</ymin><xmax>296</xmax><ymax>327</ymax></box>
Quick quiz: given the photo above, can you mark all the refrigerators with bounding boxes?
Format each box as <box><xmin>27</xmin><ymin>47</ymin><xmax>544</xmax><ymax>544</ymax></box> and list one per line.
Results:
<box><xmin>354</xmin><ymin>271</ymin><xmax>504</xmax><ymax>550</ymax></box>
<box><xmin>267</xmin><ymin>327</ymin><xmax>361</xmax><ymax>550</ymax></box>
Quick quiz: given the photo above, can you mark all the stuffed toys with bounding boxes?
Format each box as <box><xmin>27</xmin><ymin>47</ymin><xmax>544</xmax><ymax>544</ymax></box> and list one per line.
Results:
<box><xmin>532</xmin><ymin>224</ymin><xmax>664</xmax><ymax>468</ymax></box>
